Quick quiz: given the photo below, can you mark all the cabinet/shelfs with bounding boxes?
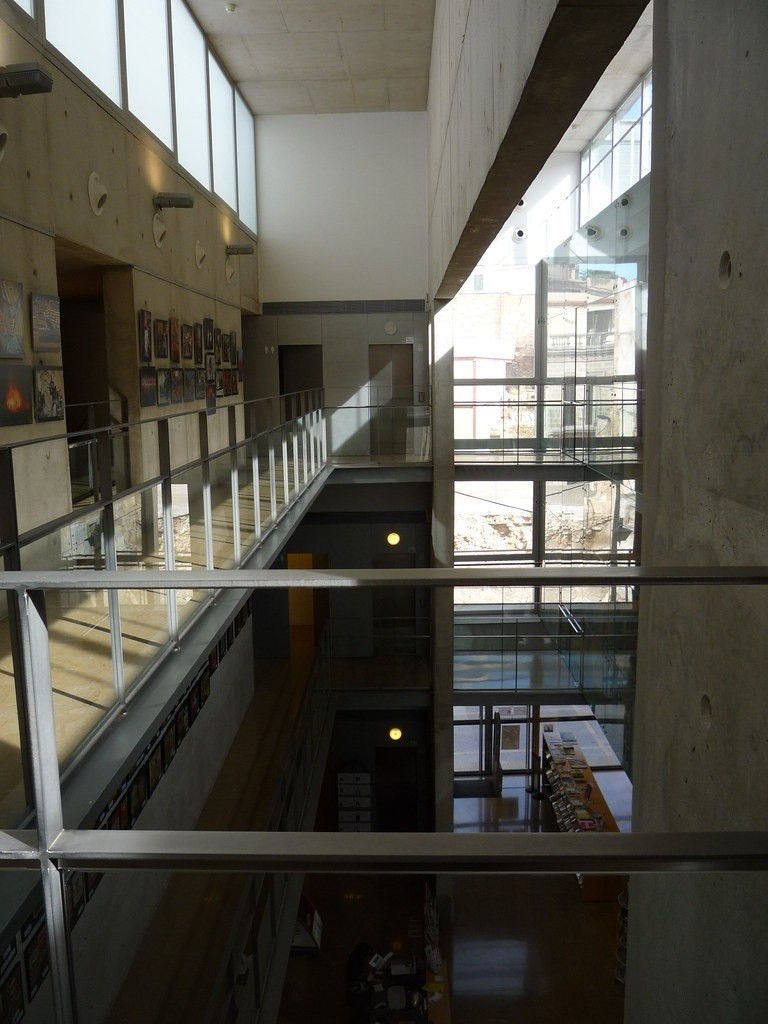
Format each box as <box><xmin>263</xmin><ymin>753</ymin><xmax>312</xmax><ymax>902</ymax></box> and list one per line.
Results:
<box><xmin>331</xmin><ymin>764</ymin><xmax>375</xmax><ymax>832</ymax></box>
<box><xmin>614</xmin><ymin>890</ymin><xmax>628</xmax><ymax>985</ymax></box>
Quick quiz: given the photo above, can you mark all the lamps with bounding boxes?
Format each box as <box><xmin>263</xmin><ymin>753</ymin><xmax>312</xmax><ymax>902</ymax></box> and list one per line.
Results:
<box><xmin>153</xmin><ymin>193</ymin><xmax>195</xmax><ymax>208</ymax></box>
<box><xmin>0</xmin><ymin>63</ymin><xmax>55</xmax><ymax>98</ymax></box>
<box><xmin>226</xmin><ymin>244</ymin><xmax>254</xmax><ymax>255</ymax></box>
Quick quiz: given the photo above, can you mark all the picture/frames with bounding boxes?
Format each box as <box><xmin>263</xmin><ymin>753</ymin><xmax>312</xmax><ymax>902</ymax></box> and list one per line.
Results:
<box><xmin>252</xmin><ymin>711</ymin><xmax>314</xmax><ymax>1008</ymax></box>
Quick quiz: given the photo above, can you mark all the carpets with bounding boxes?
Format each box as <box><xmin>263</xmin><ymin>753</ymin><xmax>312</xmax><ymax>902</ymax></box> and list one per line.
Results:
<box><xmin>453</xmin><ymin>781</ymin><xmax>498</xmax><ymax>798</ymax></box>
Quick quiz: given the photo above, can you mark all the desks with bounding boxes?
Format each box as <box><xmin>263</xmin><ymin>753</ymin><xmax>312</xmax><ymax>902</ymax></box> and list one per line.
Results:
<box><xmin>542</xmin><ymin>733</ymin><xmax>627</xmax><ymax>903</ymax></box>
<box><xmin>371</xmin><ymin>952</ymin><xmax>427</xmax><ymax>1024</ymax></box>
